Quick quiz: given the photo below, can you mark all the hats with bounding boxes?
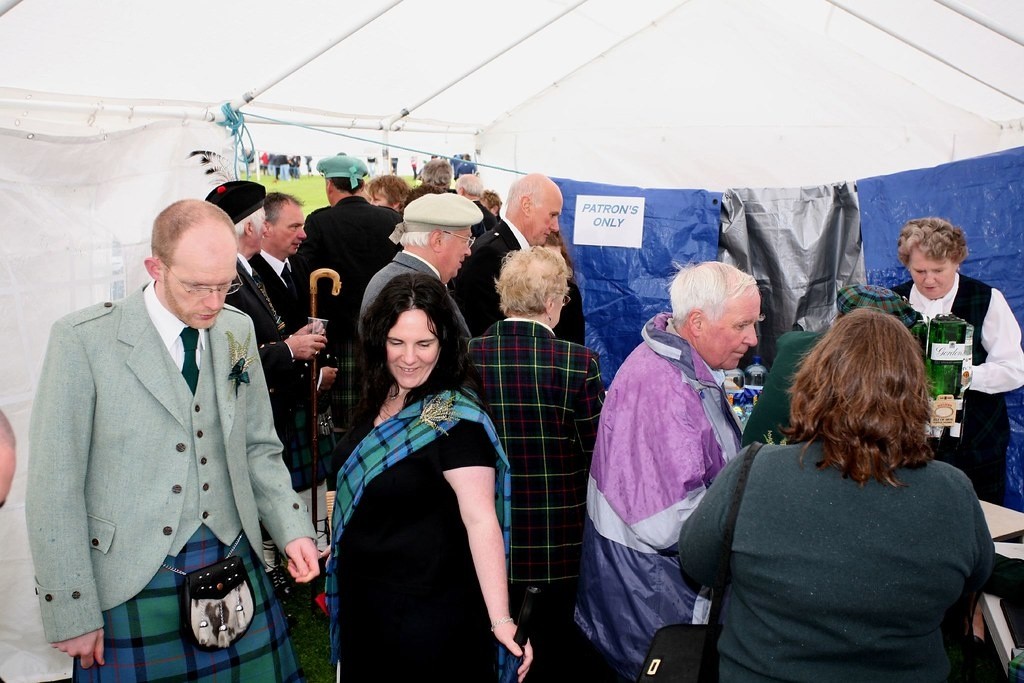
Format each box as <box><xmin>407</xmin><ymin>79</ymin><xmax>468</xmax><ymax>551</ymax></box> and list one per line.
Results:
<box><xmin>204</xmin><ymin>180</ymin><xmax>267</xmax><ymax>224</ymax></box>
<box><xmin>388</xmin><ymin>192</ymin><xmax>484</xmax><ymax>245</ymax></box>
<box><xmin>836</xmin><ymin>284</ymin><xmax>916</xmax><ymax>329</ymax></box>
<box><xmin>316</xmin><ymin>152</ymin><xmax>368</xmax><ymax>189</ymax></box>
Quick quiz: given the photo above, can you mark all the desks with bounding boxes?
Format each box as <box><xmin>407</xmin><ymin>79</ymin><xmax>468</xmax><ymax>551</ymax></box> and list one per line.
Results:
<box><xmin>980</xmin><ymin>541</ymin><xmax>1024</xmax><ymax>675</ymax></box>
<box><xmin>978</xmin><ymin>499</ymin><xmax>1024</xmax><ymax>542</ymax></box>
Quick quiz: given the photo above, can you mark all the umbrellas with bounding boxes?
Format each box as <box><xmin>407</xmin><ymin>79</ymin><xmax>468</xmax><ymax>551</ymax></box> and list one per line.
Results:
<box><xmin>499</xmin><ymin>586</ymin><xmax>543</xmax><ymax>683</ymax></box>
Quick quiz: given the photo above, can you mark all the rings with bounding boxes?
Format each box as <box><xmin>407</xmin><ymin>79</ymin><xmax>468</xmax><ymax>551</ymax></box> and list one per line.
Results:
<box><xmin>315</xmin><ymin>351</ymin><xmax>320</xmax><ymax>355</ymax></box>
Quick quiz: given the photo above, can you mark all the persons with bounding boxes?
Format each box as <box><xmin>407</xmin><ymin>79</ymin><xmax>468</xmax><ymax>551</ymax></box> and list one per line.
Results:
<box><xmin>738</xmin><ymin>279</ymin><xmax>919</xmax><ymax>449</ymax></box>
<box><xmin>675</xmin><ymin>308</ymin><xmax>998</xmax><ymax>683</ymax></box>
<box><xmin>22</xmin><ymin>197</ymin><xmax>327</xmax><ymax>683</ymax></box>
<box><xmin>885</xmin><ymin>216</ymin><xmax>1024</xmax><ymax>658</ymax></box>
<box><xmin>287</xmin><ymin>152</ymin><xmax>405</xmax><ymax>447</ymax></box>
<box><xmin>573</xmin><ymin>260</ymin><xmax>765</xmax><ymax>683</ymax></box>
<box><xmin>366</xmin><ymin>154</ymin><xmax>598</xmax><ymax>347</ymax></box>
<box><xmin>465</xmin><ymin>245</ymin><xmax>606</xmax><ymax>683</ymax></box>
<box><xmin>242</xmin><ymin>147</ymin><xmax>477</xmax><ymax>185</ymax></box>
<box><xmin>203</xmin><ymin>180</ymin><xmax>328</xmax><ymax>600</ymax></box>
<box><xmin>247</xmin><ymin>191</ymin><xmax>339</xmax><ymax>491</ymax></box>
<box><xmin>0</xmin><ymin>409</ymin><xmax>16</xmax><ymax>509</ymax></box>
<box><xmin>354</xmin><ymin>192</ymin><xmax>485</xmax><ymax>401</ymax></box>
<box><xmin>310</xmin><ymin>272</ymin><xmax>534</xmax><ymax>683</ymax></box>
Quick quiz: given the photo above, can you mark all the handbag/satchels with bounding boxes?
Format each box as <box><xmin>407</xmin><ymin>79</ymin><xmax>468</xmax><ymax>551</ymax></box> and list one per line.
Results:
<box><xmin>180</xmin><ymin>555</ymin><xmax>256</xmax><ymax>653</ymax></box>
<box><xmin>637</xmin><ymin>623</ymin><xmax>714</xmax><ymax>683</ymax></box>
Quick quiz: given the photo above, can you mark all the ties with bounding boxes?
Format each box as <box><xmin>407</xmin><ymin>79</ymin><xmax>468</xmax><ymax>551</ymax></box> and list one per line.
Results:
<box><xmin>179</xmin><ymin>326</ymin><xmax>199</xmax><ymax>396</ymax></box>
<box><xmin>252</xmin><ymin>268</ymin><xmax>263</xmax><ymax>287</ymax></box>
<box><xmin>280</xmin><ymin>263</ymin><xmax>299</xmax><ymax>299</ymax></box>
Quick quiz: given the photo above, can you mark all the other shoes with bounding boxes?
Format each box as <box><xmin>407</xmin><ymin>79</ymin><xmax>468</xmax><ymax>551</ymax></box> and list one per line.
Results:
<box><xmin>268</xmin><ymin>567</ymin><xmax>292</xmax><ymax>597</ymax></box>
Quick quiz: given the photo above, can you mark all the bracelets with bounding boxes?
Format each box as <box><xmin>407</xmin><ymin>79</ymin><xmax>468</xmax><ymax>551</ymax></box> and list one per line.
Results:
<box><xmin>487</xmin><ymin>615</ymin><xmax>515</xmax><ymax>632</ymax></box>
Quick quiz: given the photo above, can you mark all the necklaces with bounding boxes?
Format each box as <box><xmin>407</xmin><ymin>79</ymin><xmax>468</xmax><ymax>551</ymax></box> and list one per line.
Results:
<box><xmin>390</xmin><ymin>395</ymin><xmax>397</xmax><ymax>400</ymax></box>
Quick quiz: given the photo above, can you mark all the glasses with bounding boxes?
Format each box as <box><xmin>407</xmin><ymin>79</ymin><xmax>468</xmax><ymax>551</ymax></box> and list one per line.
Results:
<box><xmin>442</xmin><ymin>231</ymin><xmax>476</xmax><ymax>247</ymax></box>
<box><xmin>562</xmin><ymin>294</ymin><xmax>570</xmax><ymax>306</ymax></box>
<box><xmin>156</xmin><ymin>257</ymin><xmax>243</xmax><ymax>300</ymax></box>
